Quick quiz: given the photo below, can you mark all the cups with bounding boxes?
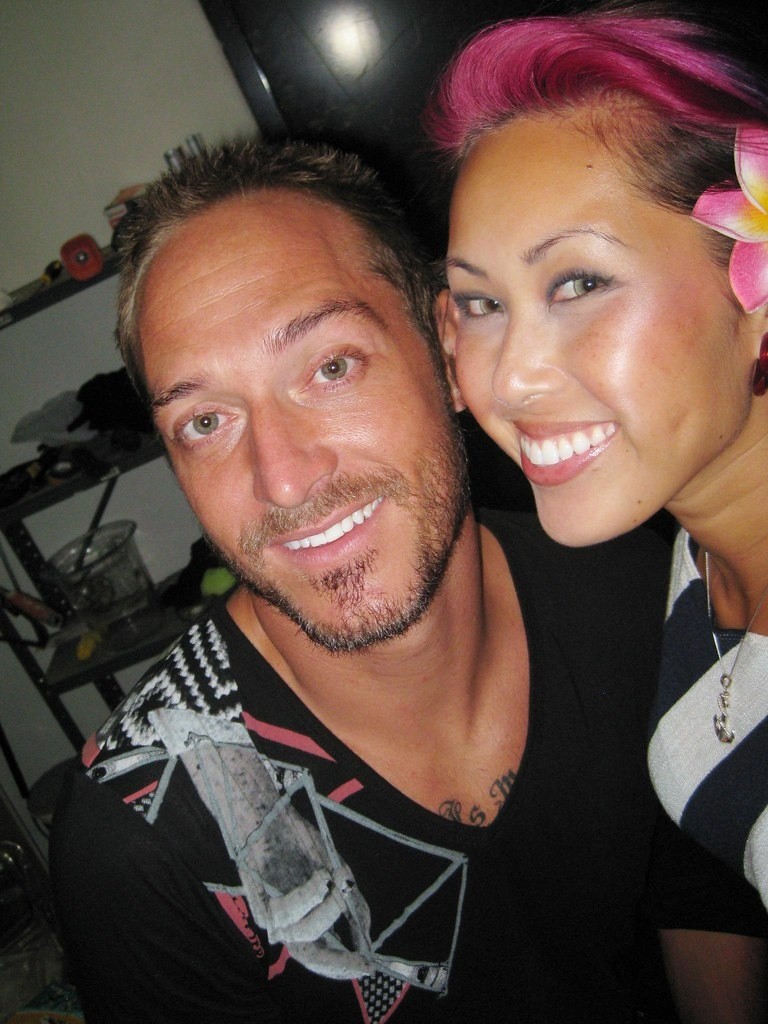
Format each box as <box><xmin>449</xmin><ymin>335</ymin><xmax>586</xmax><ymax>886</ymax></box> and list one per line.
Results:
<box><xmin>0</xmin><ymin>839</ymin><xmax>38</xmax><ymax>942</ymax></box>
<box><xmin>44</xmin><ymin>519</ymin><xmax>154</xmax><ymax>651</ymax></box>
<box><xmin>164</xmin><ymin>144</ymin><xmax>187</xmax><ymax>175</ymax></box>
<box><xmin>186</xmin><ymin>133</ymin><xmax>207</xmax><ymax>158</ymax></box>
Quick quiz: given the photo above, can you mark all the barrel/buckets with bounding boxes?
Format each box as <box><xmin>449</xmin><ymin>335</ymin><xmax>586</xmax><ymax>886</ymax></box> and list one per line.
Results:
<box><xmin>28</xmin><ymin>753</ymin><xmax>83</xmax><ymax>837</ymax></box>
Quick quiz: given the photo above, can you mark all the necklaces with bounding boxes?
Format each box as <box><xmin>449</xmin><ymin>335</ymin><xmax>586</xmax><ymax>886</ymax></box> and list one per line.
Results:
<box><xmin>702</xmin><ymin>550</ymin><xmax>768</xmax><ymax>741</ymax></box>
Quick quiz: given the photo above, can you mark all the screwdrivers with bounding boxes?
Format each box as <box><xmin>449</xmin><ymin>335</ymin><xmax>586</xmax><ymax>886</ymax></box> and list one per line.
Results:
<box><xmin>31</xmin><ymin>260</ymin><xmax>61</xmax><ymax>298</ymax></box>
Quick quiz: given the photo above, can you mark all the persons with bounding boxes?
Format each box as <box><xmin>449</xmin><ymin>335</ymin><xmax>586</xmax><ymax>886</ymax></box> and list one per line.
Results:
<box><xmin>443</xmin><ymin>14</ymin><xmax>767</xmax><ymax>918</ymax></box>
<box><xmin>1</xmin><ymin>136</ymin><xmax>768</xmax><ymax>1024</ymax></box>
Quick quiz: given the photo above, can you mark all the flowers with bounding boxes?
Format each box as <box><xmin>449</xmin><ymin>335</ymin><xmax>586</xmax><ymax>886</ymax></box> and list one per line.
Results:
<box><xmin>690</xmin><ymin>123</ymin><xmax>768</xmax><ymax>314</ymax></box>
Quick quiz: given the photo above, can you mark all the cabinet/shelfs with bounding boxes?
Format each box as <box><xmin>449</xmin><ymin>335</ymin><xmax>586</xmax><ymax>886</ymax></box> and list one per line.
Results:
<box><xmin>1</xmin><ymin>243</ymin><xmax>209</xmax><ymax>755</ymax></box>
